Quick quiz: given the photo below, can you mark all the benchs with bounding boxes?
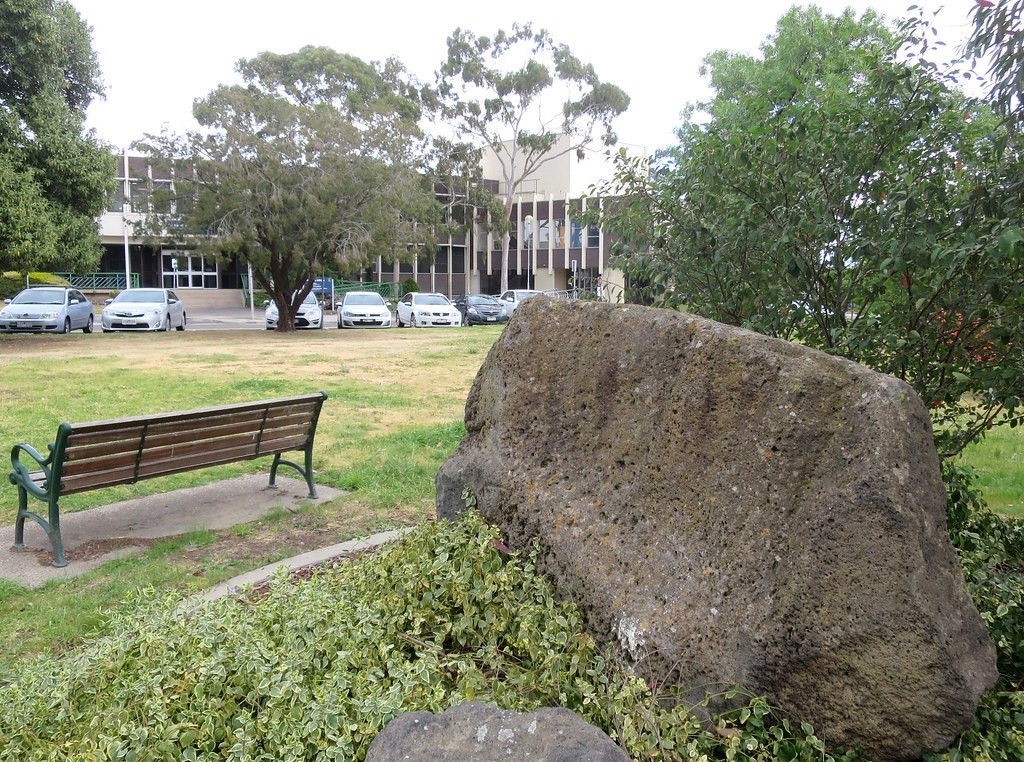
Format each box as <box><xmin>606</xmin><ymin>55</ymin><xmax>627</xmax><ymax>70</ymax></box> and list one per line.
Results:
<box><xmin>7</xmin><ymin>390</ymin><xmax>329</xmax><ymax>567</ymax></box>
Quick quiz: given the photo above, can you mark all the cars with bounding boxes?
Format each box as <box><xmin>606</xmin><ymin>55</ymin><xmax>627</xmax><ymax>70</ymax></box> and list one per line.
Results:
<box><xmin>0</xmin><ymin>286</ymin><xmax>95</xmax><ymax>335</ymax></box>
<box><xmin>501</xmin><ymin>289</ymin><xmax>546</xmax><ymax>321</ymax></box>
<box><xmin>395</xmin><ymin>292</ymin><xmax>461</xmax><ymax>328</ymax></box>
<box><xmin>492</xmin><ymin>295</ymin><xmax>501</xmax><ymax>302</ymax></box>
<box><xmin>263</xmin><ymin>289</ymin><xmax>325</xmax><ymax>330</ymax></box>
<box><xmin>455</xmin><ymin>294</ymin><xmax>509</xmax><ymax>327</ymax></box>
<box><xmin>766</xmin><ymin>301</ymin><xmax>858</xmax><ymax>322</ymax></box>
<box><xmin>101</xmin><ymin>288</ymin><xmax>186</xmax><ymax>333</ymax></box>
<box><xmin>336</xmin><ymin>291</ymin><xmax>393</xmax><ymax>329</ymax></box>
